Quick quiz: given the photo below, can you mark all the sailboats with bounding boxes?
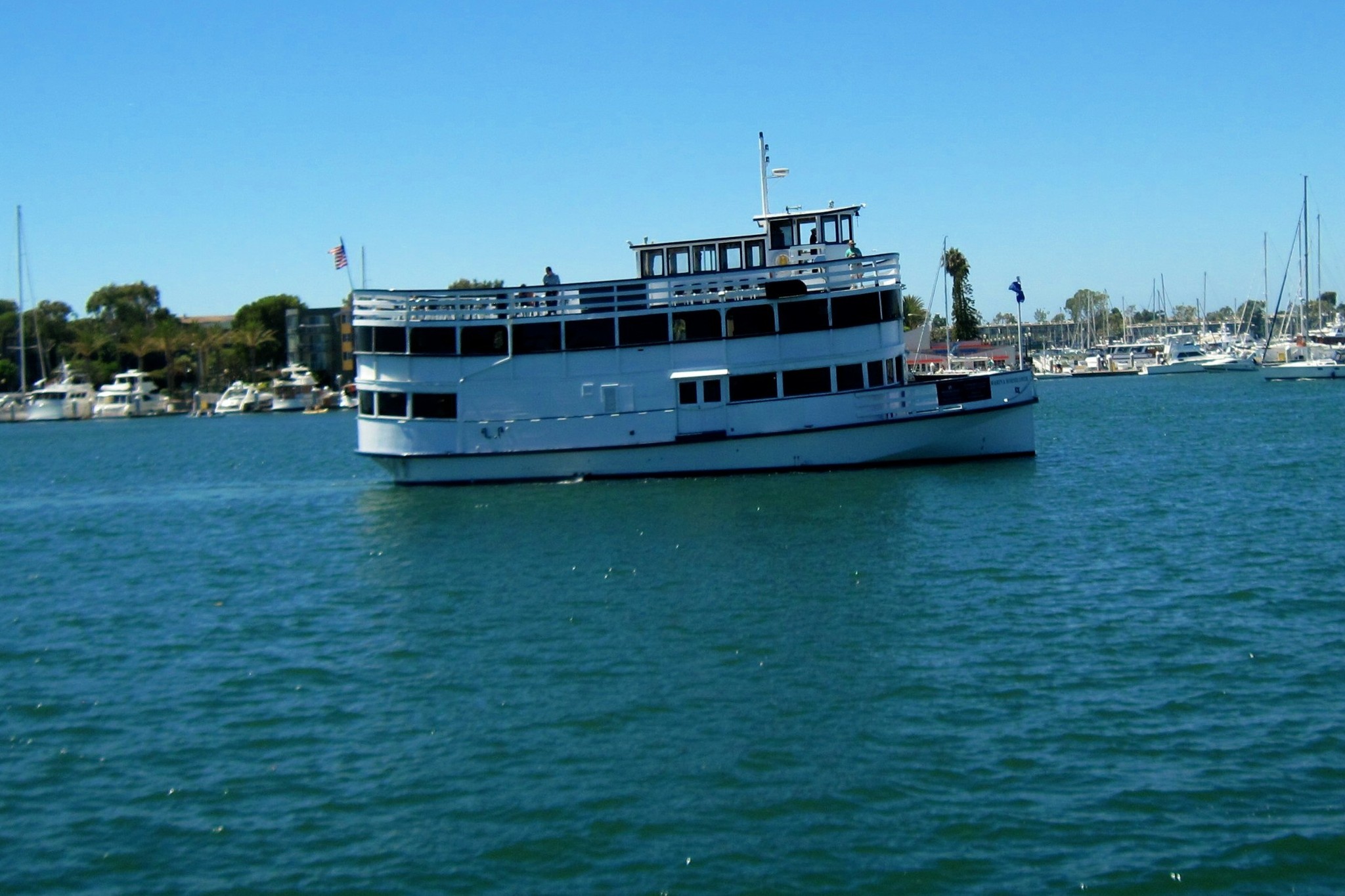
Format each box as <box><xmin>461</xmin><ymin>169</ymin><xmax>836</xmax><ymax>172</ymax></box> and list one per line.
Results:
<box><xmin>1031</xmin><ymin>174</ymin><xmax>1344</xmax><ymax>381</ymax></box>
<box><xmin>1</xmin><ymin>204</ymin><xmax>47</xmax><ymax>421</ymax></box>
<box><xmin>910</xmin><ymin>235</ymin><xmax>1025</xmax><ymax>381</ymax></box>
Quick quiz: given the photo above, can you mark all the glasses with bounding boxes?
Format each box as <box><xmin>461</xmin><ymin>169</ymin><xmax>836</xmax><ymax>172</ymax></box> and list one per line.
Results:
<box><xmin>851</xmin><ymin>244</ymin><xmax>855</xmax><ymax>245</ymax></box>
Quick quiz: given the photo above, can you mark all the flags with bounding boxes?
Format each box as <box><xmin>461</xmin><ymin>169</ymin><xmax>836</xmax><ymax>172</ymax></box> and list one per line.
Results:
<box><xmin>1008</xmin><ymin>281</ymin><xmax>1025</xmax><ymax>303</ymax></box>
<box><xmin>328</xmin><ymin>246</ymin><xmax>347</xmax><ymax>270</ymax></box>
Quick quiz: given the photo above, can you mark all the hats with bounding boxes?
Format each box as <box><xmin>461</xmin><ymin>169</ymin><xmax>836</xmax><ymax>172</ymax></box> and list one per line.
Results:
<box><xmin>848</xmin><ymin>240</ymin><xmax>856</xmax><ymax>245</ymax></box>
<box><xmin>810</xmin><ymin>228</ymin><xmax>816</xmax><ymax>232</ymax></box>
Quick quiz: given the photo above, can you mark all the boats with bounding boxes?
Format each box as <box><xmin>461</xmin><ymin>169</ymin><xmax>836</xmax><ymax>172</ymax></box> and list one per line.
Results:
<box><xmin>93</xmin><ymin>370</ymin><xmax>170</xmax><ymax>419</ymax></box>
<box><xmin>349</xmin><ymin>130</ymin><xmax>1040</xmax><ymax>487</ymax></box>
<box><xmin>26</xmin><ymin>357</ymin><xmax>96</xmax><ymax>421</ymax></box>
<box><xmin>271</xmin><ymin>361</ymin><xmax>359</xmax><ymax>413</ymax></box>
<box><xmin>214</xmin><ymin>382</ymin><xmax>275</xmax><ymax>414</ymax></box>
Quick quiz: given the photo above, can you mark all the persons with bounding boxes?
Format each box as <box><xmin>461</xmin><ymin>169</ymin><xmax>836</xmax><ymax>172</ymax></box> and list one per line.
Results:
<box><xmin>846</xmin><ymin>240</ymin><xmax>865</xmax><ymax>289</ymax></box>
<box><xmin>810</xmin><ymin>228</ymin><xmax>818</xmax><ymax>273</ymax></box>
<box><xmin>543</xmin><ymin>267</ymin><xmax>560</xmax><ymax>316</ymax></box>
<box><xmin>518</xmin><ymin>284</ymin><xmax>535</xmax><ymax>317</ymax></box>
